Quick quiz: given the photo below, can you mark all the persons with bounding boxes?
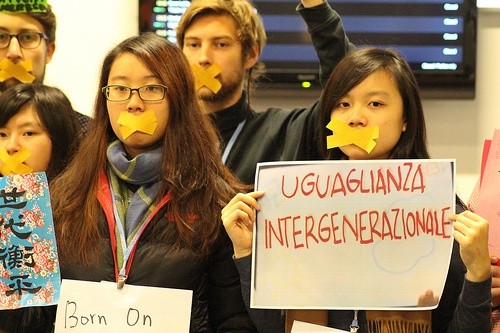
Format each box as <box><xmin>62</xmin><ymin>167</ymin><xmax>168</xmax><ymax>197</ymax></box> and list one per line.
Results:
<box><xmin>220</xmin><ymin>48</ymin><xmax>492</xmax><ymax>333</ymax></box>
<box><xmin>0</xmin><ymin>0</ymin><xmax>500</xmax><ymax>333</ymax></box>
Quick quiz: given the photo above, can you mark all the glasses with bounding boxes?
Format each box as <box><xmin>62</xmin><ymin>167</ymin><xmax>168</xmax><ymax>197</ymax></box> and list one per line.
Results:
<box><xmin>101</xmin><ymin>82</ymin><xmax>167</xmax><ymax>102</ymax></box>
<box><xmin>0</xmin><ymin>32</ymin><xmax>47</xmax><ymax>49</ymax></box>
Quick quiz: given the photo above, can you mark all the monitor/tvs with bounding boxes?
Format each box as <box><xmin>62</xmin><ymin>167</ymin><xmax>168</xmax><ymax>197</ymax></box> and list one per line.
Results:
<box><xmin>138</xmin><ymin>0</ymin><xmax>477</xmax><ymax>87</ymax></box>
